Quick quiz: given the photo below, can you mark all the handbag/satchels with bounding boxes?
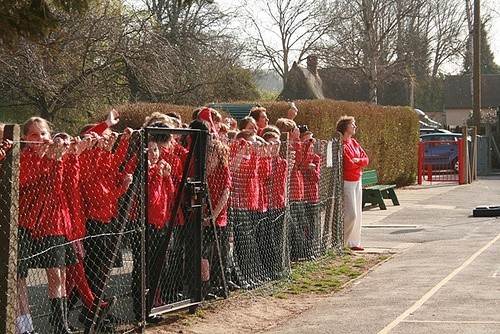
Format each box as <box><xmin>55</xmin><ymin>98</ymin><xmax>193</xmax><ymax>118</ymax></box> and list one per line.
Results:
<box><xmin>201</xmin><ymin>259</ymin><xmax>209</xmax><ymax>281</ymax></box>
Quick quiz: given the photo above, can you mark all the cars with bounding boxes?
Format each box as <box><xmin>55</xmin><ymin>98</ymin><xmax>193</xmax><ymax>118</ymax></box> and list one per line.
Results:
<box><xmin>418</xmin><ymin>133</ymin><xmax>472</xmax><ymax>174</ymax></box>
<box><xmin>415</xmin><ymin>108</ymin><xmax>451</xmax><ymax>134</ymax></box>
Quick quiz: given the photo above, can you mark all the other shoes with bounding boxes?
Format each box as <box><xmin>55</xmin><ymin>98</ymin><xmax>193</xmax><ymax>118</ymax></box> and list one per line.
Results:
<box><xmin>207</xmin><ymin>287</ymin><xmax>223</xmax><ymax>299</ymax></box>
<box><xmin>351</xmin><ymin>246</ymin><xmax>364</xmax><ymax>251</ymax></box>
<box><xmin>226</xmin><ymin>280</ymin><xmax>240</xmax><ymax>292</ymax></box>
<box><xmin>97</xmin><ymin>296</ymin><xmax>117</xmax><ymax>324</ymax></box>
<box><xmin>67</xmin><ymin>321</ymin><xmax>78</xmax><ymax>331</ymax></box>
<box><xmin>242</xmin><ymin>277</ymin><xmax>270</xmax><ymax>290</ymax></box>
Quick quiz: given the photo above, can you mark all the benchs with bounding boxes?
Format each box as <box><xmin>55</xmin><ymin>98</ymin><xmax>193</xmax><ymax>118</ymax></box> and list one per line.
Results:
<box><xmin>360</xmin><ymin>169</ymin><xmax>400</xmax><ymax>211</ymax></box>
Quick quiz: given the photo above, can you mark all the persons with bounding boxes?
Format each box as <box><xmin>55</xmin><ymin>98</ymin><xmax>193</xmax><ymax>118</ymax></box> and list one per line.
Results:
<box><xmin>0</xmin><ymin>104</ymin><xmax>321</xmax><ymax>334</ymax></box>
<box><xmin>336</xmin><ymin>114</ymin><xmax>369</xmax><ymax>250</ymax></box>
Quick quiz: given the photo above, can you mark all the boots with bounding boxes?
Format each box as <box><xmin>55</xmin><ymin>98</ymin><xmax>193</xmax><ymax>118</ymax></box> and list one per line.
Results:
<box><xmin>49</xmin><ymin>297</ymin><xmax>79</xmax><ymax>334</ymax></box>
